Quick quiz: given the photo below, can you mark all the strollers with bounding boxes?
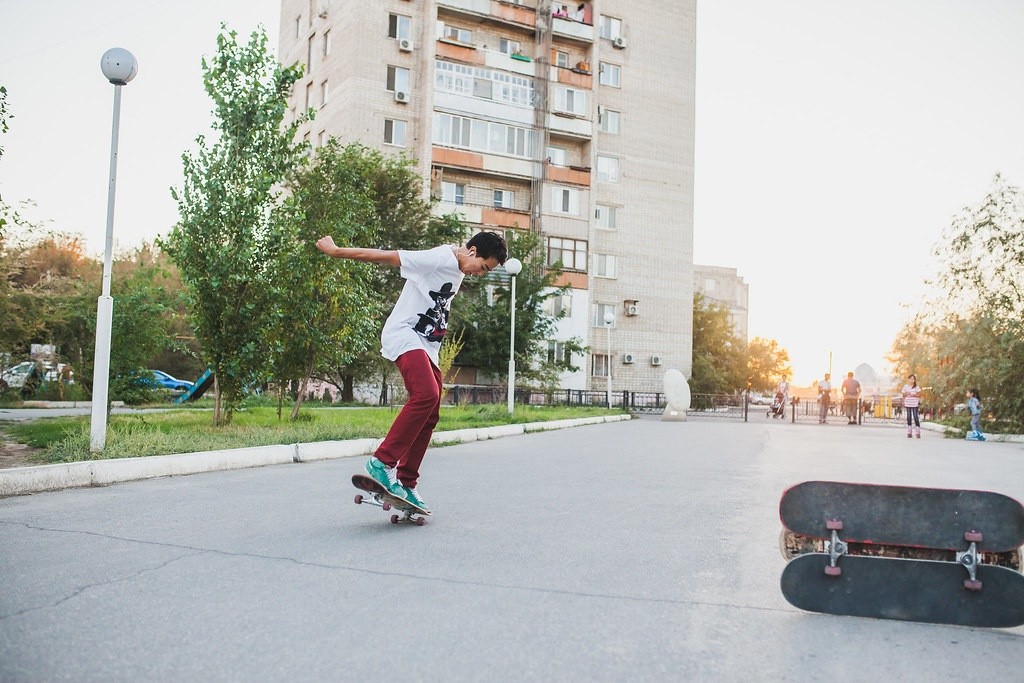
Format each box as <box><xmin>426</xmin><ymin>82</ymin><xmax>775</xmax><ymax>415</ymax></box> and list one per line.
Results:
<box><xmin>766</xmin><ymin>392</ymin><xmax>787</xmax><ymax>420</ymax></box>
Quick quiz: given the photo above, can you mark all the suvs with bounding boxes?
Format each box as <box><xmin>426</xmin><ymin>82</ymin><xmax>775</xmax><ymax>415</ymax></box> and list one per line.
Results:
<box><xmin>0</xmin><ymin>361</ymin><xmax>75</xmax><ymax>396</ymax></box>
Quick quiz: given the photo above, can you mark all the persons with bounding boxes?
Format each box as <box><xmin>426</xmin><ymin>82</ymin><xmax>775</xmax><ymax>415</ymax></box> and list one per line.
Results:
<box><xmin>771</xmin><ymin>398</ymin><xmax>781</xmax><ymax>408</ymax></box>
<box><xmin>901</xmin><ymin>375</ymin><xmax>922</xmax><ymax>439</ymax></box>
<box><xmin>841</xmin><ymin>372</ymin><xmax>861</xmax><ymax>425</ymax></box>
<box><xmin>962</xmin><ymin>388</ymin><xmax>985</xmax><ymax>441</ymax></box>
<box><xmin>818</xmin><ymin>373</ymin><xmax>832</xmax><ymax>424</ymax></box>
<box><xmin>778</xmin><ymin>374</ymin><xmax>790</xmax><ymax>417</ymax></box>
<box><xmin>316</xmin><ymin>232</ymin><xmax>508</xmax><ymax>514</ymax></box>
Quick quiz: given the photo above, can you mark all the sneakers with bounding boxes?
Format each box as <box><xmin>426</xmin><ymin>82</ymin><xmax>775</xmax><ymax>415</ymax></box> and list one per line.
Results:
<box><xmin>401</xmin><ymin>482</ymin><xmax>429</xmax><ymax>513</ymax></box>
<box><xmin>365</xmin><ymin>455</ymin><xmax>407</xmax><ymax>498</ymax></box>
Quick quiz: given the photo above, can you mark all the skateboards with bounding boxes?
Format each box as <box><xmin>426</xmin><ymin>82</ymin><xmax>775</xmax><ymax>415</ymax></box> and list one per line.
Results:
<box><xmin>779</xmin><ymin>481</ymin><xmax>1024</xmax><ymax>629</ymax></box>
<box><xmin>351</xmin><ymin>474</ymin><xmax>434</xmax><ymax>526</ymax></box>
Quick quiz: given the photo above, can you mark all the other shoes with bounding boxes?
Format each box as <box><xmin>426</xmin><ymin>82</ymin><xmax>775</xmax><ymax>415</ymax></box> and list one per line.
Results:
<box><xmin>971</xmin><ymin>431</ymin><xmax>984</xmax><ymax>440</ymax></box>
<box><xmin>848</xmin><ymin>421</ymin><xmax>857</xmax><ymax>424</ymax></box>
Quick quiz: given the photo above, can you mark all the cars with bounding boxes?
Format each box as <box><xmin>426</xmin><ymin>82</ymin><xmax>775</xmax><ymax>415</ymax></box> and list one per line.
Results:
<box><xmin>116</xmin><ymin>368</ymin><xmax>195</xmax><ymax>397</ymax></box>
<box><xmin>751</xmin><ymin>393</ymin><xmax>777</xmax><ymax>405</ymax></box>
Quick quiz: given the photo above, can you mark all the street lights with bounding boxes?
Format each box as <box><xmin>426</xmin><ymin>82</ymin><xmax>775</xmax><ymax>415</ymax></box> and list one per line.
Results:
<box><xmin>504</xmin><ymin>257</ymin><xmax>523</xmax><ymax>414</ymax></box>
<box><xmin>603</xmin><ymin>312</ymin><xmax>615</xmax><ymax>408</ymax></box>
<box><xmin>86</xmin><ymin>45</ymin><xmax>142</xmax><ymax>453</ymax></box>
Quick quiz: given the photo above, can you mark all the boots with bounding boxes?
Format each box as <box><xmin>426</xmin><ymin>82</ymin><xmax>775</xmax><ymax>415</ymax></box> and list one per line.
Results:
<box><xmin>915</xmin><ymin>426</ymin><xmax>920</xmax><ymax>437</ymax></box>
<box><xmin>907</xmin><ymin>425</ymin><xmax>912</xmax><ymax>438</ymax></box>
